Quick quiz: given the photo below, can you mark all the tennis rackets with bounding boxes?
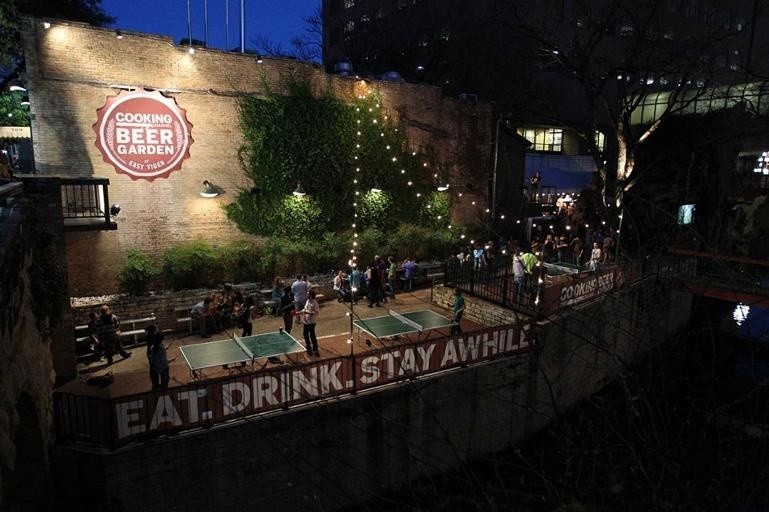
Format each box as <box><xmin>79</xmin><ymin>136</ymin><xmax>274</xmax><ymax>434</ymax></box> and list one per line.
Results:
<box><xmin>446</xmin><ymin>303</ymin><xmax>451</xmax><ymax>308</ymax></box>
<box><xmin>291</xmin><ymin>310</ymin><xmax>299</xmax><ymax>316</ymax></box>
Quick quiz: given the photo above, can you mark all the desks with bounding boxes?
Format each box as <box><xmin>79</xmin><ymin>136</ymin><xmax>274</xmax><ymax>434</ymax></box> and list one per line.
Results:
<box><xmin>353</xmin><ymin>308</ymin><xmax>460</xmax><ymax>353</ymax></box>
<box><xmin>179</xmin><ymin>329</ymin><xmax>307</xmax><ymax>382</ymax></box>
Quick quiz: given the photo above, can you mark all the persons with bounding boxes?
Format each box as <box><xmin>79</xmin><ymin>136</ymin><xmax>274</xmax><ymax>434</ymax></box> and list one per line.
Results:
<box><xmin>450</xmin><ymin>192</ymin><xmax>617</xmax><ymax>306</ymax></box>
<box><xmin>272</xmin><ymin>273</ymin><xmax>321</xmax><ymax>355</ymax></box>
<box><xmin>190</xmin><ymin>283</ymin><xmax>254</xmax><ymax>337</ymax></box>
<box><xmin>144</xmin><ymin>325</ymin><xmax>174</xmax><ymax>389</ymax></box>
<box><xmin>87</xmin><ymin>305</ymin><xmax>132</xmax><ymax>364</ymax></box>
<box><xmin>452</xmin><ymin>287</ymin><xmax>466</xmax><ymax>337</ymax></box>
<box><xmin>333</xmin><ymin>255</ymin><xmax>416</xmax><ymax>308</ymax></box>
<box><xmin>530</xmin><ymin>169</ymin><xmax>541</xmax><ymax>202</ymax></box>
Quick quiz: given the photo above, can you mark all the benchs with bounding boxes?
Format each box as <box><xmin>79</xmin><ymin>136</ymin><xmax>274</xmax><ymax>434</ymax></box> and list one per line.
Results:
<box><xmin>74</xmin><ymin>316</ymin><xmax>157</xmax><ymax>346</ymax></box>
<box><xmin>174</xmin><ymin>284</ymin><xmax>324</xmax><ymax>335</ymax></box>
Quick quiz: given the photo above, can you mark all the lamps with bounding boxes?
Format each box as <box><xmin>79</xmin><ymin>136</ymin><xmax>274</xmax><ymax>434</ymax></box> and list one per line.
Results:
<box><xmin>200</xmin><ymin>181</ymin><xmax>218</xmax><ymax>198</ymax></box>
<box><xmin>292</xmin><ymin>181</ymin><xmax>307</xmax><ymax>196</ymax></box>
